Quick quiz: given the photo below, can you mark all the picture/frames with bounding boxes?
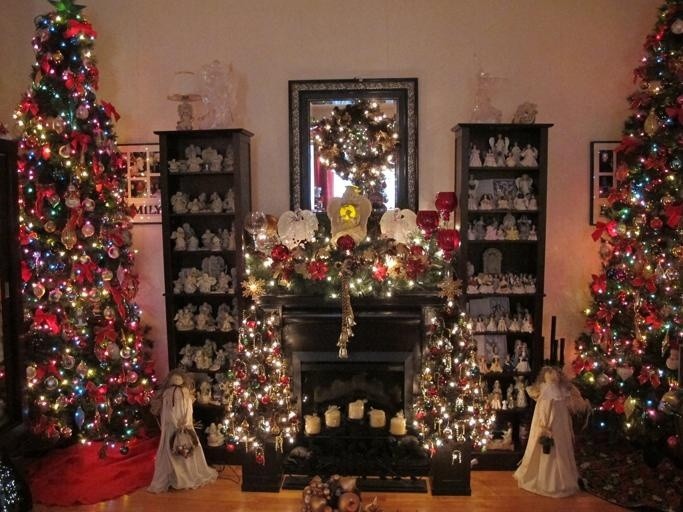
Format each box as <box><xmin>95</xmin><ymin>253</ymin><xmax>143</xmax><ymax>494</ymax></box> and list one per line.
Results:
<box><xmin>116</xmin><ymin>143</ymin><xmax>163</xmax><ymax>225</ymax></box>
<box><xmin>590</xmin><ymin>141</ymin><xmax>622</xmax><ymax>225</ymax></box>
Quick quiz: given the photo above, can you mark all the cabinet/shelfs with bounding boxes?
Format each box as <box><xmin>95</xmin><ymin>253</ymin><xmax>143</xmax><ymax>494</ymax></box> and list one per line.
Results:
<box><xmin>153</xmin><ymin>128</ymin><xmax>255</xmax><ymax>466</ymax></box>
<box><xmin>450</xmin><ymin>124</ymin><xmax>554</xmax><ymax>471</ymax></box>
<box><xmin>0</xmin><ymin>138</ymin><xmax>60</xmax><ymax>460</ymax></box>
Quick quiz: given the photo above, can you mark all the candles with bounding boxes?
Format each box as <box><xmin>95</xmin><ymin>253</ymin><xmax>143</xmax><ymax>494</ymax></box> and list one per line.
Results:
<box><xmin>389</xmin><ymin>410</ymin><xmax>408</xmax><ymax>436</ymax></box>
<box><xmin>368</xmin><ymin>407</ymin><xmax>386</xmax><ymax>428</ymax></box>
<box><xmin>349</xmin><ymin>399</ymin><xmax>368</xmax><ymax>419</ymax></box>
<box><xmin>304</xmin><ymin>413</ymin><xmax>320</xmax><ymax>435</ymax></box>
<box><xmin>325</xmin><ymin>405</ymin><xmax>341</xmax><ymax>428</ymax></box>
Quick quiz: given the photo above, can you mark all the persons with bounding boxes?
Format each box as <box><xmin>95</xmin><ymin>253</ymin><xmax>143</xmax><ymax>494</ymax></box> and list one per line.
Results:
<box><xmin>147</xmin><ymin>367</ymin><xmax>219</xmax><ymax>494</ymax></box>
<box><xmin>462</xmin><ymin>135</ymin><xmax>539</xmax><ymax>448</ymax></box>
<box><xmin>512</xmin><ymin>363</ymin><xmax>587</xmax><ymax>498</ymax></box>
<box><xmin>167</xmin><ymin>144</ymin><xmax>238</xmax><ymax>447</ymax></box>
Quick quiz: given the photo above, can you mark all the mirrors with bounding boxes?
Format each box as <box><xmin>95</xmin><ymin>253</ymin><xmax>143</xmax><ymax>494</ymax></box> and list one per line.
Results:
<box><xmin>288</xmin><ymin>77</ymin><xmax>419</xmax><ymax>213</ymax></box>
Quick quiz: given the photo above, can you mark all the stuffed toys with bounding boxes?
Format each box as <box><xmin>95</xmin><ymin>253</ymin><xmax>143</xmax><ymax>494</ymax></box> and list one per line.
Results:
<box><xmin>327</xmin><ymin>196</ymin><xmax>372</xmax><ymax>246</ymax></box>
<box><xmin>379</xmin><ymin>208</ymin><xmax>418</xmax><ymax>245</ymax></box>
<box><xmin>274</xmin><ymin>209</ymin><xmax>317</xmax><ymax>245</ymax></box>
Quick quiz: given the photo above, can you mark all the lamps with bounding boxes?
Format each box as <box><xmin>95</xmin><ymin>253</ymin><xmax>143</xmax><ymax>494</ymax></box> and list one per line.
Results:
<box><xmin>167</xmin><ymin>72</ymin><xmax>202</xmax><ymax>130</ymax></box>
